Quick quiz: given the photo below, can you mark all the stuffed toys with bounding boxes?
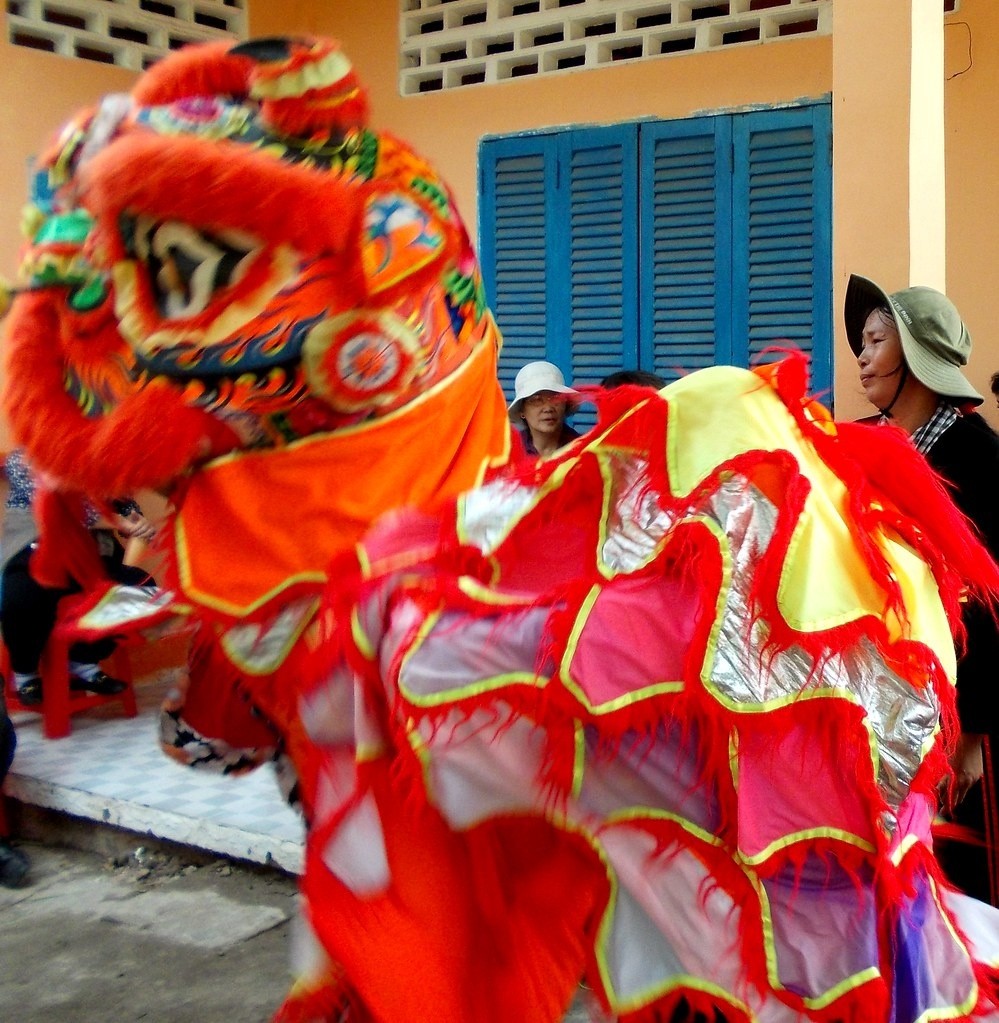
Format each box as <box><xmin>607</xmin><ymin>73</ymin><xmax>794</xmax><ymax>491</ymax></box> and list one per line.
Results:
<box><xmin>1</xmin><ymin>33</ymin><xmax>999</xmax><ymax>1023</ymax></box>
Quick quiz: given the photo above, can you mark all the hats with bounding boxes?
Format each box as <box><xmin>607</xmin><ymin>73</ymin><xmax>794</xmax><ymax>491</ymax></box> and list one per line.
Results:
<box><xmin>507</xmin><ymin>360</ymin><xmax>581</xmax><ymax>423</ymax></box>
<box><xmin>843</xmin><ymin>272</ymin><xmax>987</xmax><ymax>411</ymax></box>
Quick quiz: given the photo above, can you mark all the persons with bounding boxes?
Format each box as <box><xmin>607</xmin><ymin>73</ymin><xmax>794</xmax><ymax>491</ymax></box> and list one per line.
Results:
<box><xmin>601</xmin><ymin>370</ymin><xmax>666</xmax><ymax>390</ymax></box>
<box><xmin>0</xmin><ymin>449</ymin><xmax>155</xmax><ymax>704</ymax></box>
<box><xmin>507</xmin><ymin>361</ymin><xmax>584</xmax><ymax>457</ymax></box>
<box><xmin>0</xmin><ymin>672</ymin><xmax>31</xmax><ymax>887</ymax></box>
<box><xmin>843</xmin><ymin>273</ymin><xmax>999</xmax><ymax>908</ymax></box>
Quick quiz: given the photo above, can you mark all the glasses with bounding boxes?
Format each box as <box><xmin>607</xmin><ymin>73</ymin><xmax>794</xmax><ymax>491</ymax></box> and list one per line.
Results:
<box><xmin>525</xmin><ymin>395</ymin><xmax>569</xmax><ymax>406</ymax></box>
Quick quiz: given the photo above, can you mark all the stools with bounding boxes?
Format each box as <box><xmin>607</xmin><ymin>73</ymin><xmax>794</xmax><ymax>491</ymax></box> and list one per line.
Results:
<box><xmin>0</xmin><ymin>628</ymin><xmax>137</xmax><ymax>739</ymax></box>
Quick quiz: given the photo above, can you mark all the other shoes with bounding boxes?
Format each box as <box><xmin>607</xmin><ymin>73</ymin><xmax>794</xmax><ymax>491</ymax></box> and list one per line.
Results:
<box><xmin>0</xmin><ymin>838</ymin><xmax>34</xmax><ymax>888</ymax></box>
<box><xmin>15</xmin><ymin>677</ymin><xmax>44</xmax><ymax>706</ymax></box>
<box><xmin>71</xmin><ymin>669</ymin><xmax>130</xmax><ymax>697</ymax></box>
